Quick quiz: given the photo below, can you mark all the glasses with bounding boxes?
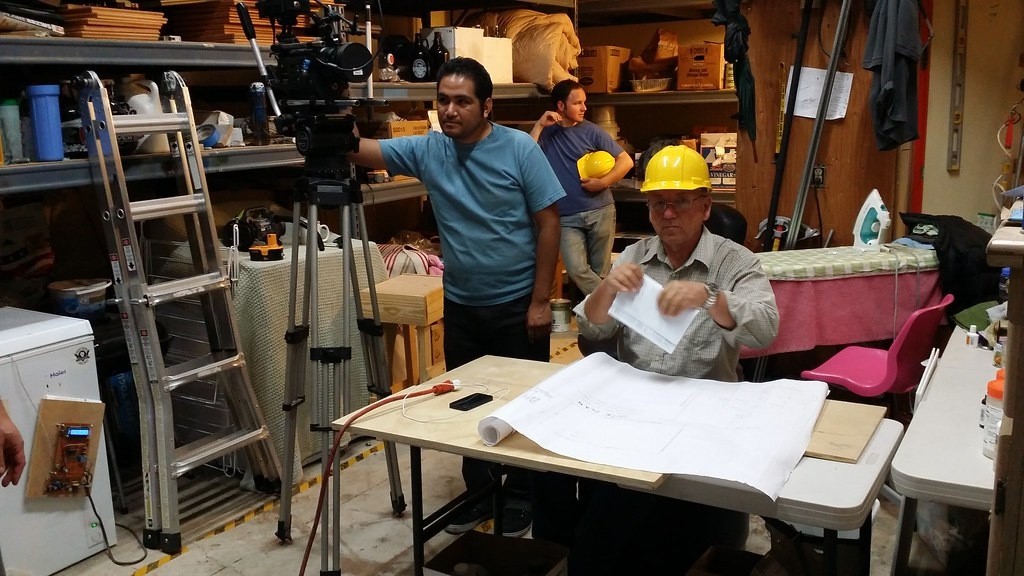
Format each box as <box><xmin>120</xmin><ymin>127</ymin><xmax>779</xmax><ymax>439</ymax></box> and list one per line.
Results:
<box><xmin>646</xmin><ymin>192</ymin><xmax>703</xmax><ymax>211</ymax></box>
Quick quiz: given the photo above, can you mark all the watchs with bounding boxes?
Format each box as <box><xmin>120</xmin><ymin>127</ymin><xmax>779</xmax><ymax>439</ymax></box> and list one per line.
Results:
<box><xmin>701</xmin><ymin>283</ymin><xmax>719</xmax><ymax>309</ymax></box>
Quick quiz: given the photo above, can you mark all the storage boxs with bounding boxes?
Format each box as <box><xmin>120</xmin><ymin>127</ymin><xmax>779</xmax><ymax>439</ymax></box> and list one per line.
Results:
<box><xmin>423</xmin><ymin>529</ymin><xmax>569</xmax><ymax>576</ymax></box>
<box><xmin>576</xmin><ymin>46</ymin><xmax>630</xmax><ymax>93</ymax></box>
<box><xmin>359</xmin><ymin>121</ymin><xmax>428</xmax><ymax>182</ymax></box>
<box><xmin>677</xmin><ymin>39</ymin><xmax>727</xmax><ymax>90</ymax></box>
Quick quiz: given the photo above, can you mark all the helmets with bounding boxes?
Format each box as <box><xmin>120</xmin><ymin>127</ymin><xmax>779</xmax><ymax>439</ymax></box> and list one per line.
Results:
<box><xmin>641</xmin><ymin>144</ymin><xmax>712</xmax><ymax>192</ymax></box>
<box><xmin>577</xmin><ymin>151</ymin><xmax>616</xmax><ymax>179</ymax></box>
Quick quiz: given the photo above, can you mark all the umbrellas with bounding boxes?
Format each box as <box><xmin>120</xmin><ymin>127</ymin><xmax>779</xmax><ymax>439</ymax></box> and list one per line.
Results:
<box><xmin>712</xmin><ymin>0</ymin><xmax>758</xmax><ymax>164</ymax></box>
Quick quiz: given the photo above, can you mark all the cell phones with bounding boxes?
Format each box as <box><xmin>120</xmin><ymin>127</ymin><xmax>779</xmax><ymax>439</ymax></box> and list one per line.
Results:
<box><xmin>450</xmin><ymin>393</ymin><xmax>494</xmax><ymax>411</ymax></box>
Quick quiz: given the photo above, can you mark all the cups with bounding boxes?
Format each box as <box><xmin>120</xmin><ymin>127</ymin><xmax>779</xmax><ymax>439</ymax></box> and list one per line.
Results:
<box><xmin>27</xmin><ymin>84</ymin><xmax>65</xmax><ymax>162</ymax></box>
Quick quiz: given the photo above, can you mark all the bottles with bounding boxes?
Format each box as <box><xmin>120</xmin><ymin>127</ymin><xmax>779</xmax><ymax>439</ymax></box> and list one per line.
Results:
<box><xmin>428</xmin><ymin>31</ymin><xmax>450</xmax><ymax>83</ymax></box>
<box><xmin>982</xmin><ymin>369</ymin><xmax>1005</xmax><ymax>460</ymax></box>
<box><xmin>979</xmin><ymin>394</ymin><xmax>987</xmax><ymax>429</ymax></box>
<box><xmin>407</xmin><ymin>32</ymin><xmax>431</xmax><ymax>83</ymax></box>
<box><xmin>965</xmin><ymin>325</ymin><xmax>979</xmax><ymax>348</ymax></box>
<box><xmin>248</xmin><ymin>80</ymin><xmax>270</xmax><ymax>146</ymax></box>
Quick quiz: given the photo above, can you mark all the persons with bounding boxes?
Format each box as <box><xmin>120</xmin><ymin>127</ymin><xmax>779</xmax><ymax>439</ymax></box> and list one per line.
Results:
<box><xmin>340</xmin><ymin>56</ymin><xmax>568</xmax><ymax>537</ymax></box>
<box><xmin>530</xmin><ymin>79</ymin><xmax>634</xmax><ymax>296</ymax></box>
<box><xmin>573</xmin><ymin>144</ymin><xmax>779</xmax><ymax>576</ymax></box>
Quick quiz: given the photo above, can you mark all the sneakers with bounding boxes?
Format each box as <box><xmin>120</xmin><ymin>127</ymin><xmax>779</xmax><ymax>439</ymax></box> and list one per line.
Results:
<box><xmin>502</xmin><ymin>509</ymin><xmax>533</xmax><ymax>536</ymax></box>
<box><xmin>444</xmin><ymin>510</ymin><xmax>494</xmax><ymax>533</ymax></box>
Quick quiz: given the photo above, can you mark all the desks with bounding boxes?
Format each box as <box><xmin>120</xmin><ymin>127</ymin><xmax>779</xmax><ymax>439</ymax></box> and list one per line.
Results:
<box><xmin>891</xmin><ymin>323</ymin><xmax>1008</xmax><ymax>576</ymax></box>
<box><xmin>331</xmin><ymin>355</ymin><xmax>904</xmax><ymax>576</ymax></box>
<box><xmin>739</xmin><ymin>244</ymin><xmax>941</xmax><ymax>383</ymax></box>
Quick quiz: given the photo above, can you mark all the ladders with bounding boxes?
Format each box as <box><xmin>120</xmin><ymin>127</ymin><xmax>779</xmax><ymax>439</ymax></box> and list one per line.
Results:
<box><xmin>69</xmin><ymin>68</ymin><xmax>286</xmax><ymax>559</ymax></box>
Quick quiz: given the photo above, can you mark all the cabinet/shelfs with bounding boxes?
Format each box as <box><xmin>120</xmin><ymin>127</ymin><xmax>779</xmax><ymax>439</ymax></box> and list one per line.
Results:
<box><xmin>0</xmin><ymin>0</ymin><xmax>738</xmax><ymax>386</ymax></box>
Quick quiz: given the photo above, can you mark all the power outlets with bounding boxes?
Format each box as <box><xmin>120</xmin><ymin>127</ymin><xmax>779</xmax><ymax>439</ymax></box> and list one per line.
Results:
<box><xmin>810</xmin><ymin>166</ymin><xmax>825</xmax><ymax>188</ymax></box>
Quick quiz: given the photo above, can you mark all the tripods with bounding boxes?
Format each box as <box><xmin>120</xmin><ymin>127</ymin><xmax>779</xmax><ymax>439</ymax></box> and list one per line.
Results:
<box><xmin>234</xmin><ymin>2</ymin><xmax>408</xmax><ymax>544</ymax></box>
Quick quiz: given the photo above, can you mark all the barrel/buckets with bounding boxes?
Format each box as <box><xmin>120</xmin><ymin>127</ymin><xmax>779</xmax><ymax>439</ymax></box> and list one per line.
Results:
<box><xmin>550</xmin><ymin>298</ymin><xmax>572</xmax><ymax>332</ymax></box>
<box><xmin>47</xmin><ymin>278</ymin><xmax>113</xmax><ymax>315</ymax></box>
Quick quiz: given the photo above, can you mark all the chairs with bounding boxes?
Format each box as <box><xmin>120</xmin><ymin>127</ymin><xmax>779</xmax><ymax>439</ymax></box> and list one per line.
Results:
<box><xmin>801</xmin><ymin>292</ymin><xmax>954</xmax><ymax>420</ymax></box>
